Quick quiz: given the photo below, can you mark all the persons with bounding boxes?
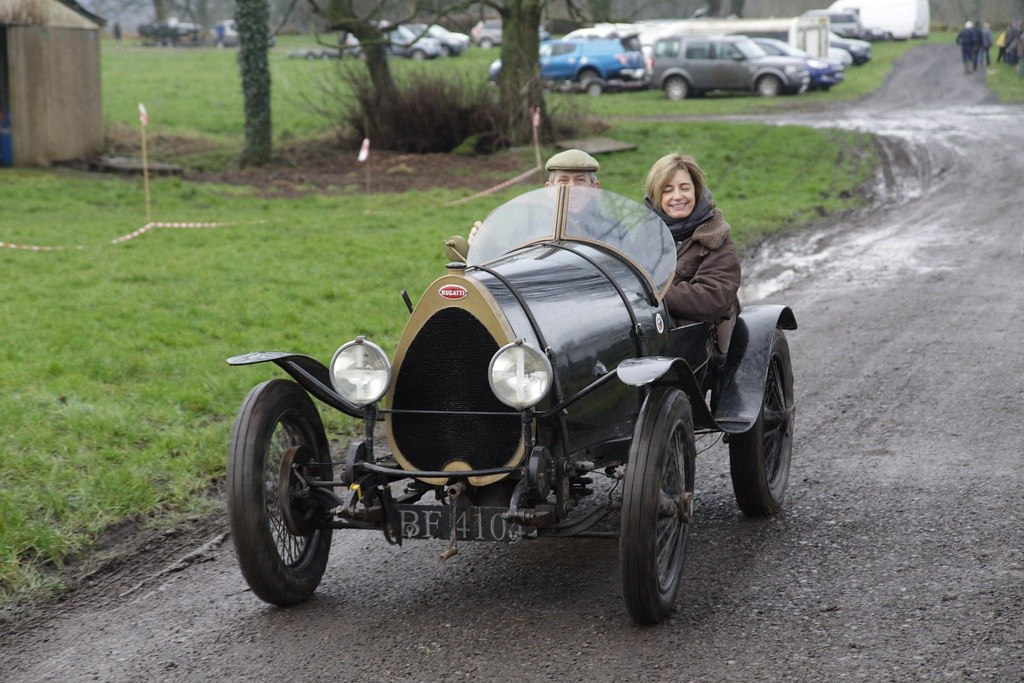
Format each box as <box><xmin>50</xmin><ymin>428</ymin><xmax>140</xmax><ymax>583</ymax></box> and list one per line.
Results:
<box><xmin>957</xmin><ymin>20</ymin><xmax>992</xmax><ymax>74</ymax></box>
<box><xmin>468</xmin><ymin>148</ymin><xmax>630</xmax><ymax>251</ymax></box>
<box><xmin>995</xmin><ymin>19</ymin><xmax>1024</xmax><ymax>76</ymax></box>
<box><xmin>634</xmin><ymin>154</ymin><xmax>741</xmax><ymax>325</ymax></box>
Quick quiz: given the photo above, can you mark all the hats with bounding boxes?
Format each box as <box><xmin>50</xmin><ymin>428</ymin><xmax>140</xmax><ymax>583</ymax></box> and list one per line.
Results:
<box><xmin>545</xmin><ymin>149</ymin><xmax>600</xmax><ymax>170</ymax></box>
<box><xmin>965</xmin><ymin>21</ymin><xmax>973</xmax><ymax>28</ymax></box>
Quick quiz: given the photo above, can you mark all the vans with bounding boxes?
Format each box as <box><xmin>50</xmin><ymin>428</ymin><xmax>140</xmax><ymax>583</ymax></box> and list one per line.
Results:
<box><xmin>489</xmin><ymin>33</ymin><xmax>643</xmax><ymax>95</ymax></box>
<box><xmin>564</xmin><ymin>20</ymin><xmax>651</xmax><ymax>83</ymax></box>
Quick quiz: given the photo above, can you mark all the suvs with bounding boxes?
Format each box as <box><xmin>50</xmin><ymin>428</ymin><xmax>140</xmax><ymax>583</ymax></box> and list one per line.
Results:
<box><xmin>649</xmin><ymin>34</ymin><xmax>809</xmax><ymax>100</ymax></box>
<box><xmin>830</xmin><ymin>31</ymin><xmax>870</xmax><ymax>66</ymax></box>
<box><xmin>340</xmin><ymin>20</ymin><xmax>448</xmax><ymax>58</ymax></box>
<box><xmin>798</xmin><ymin>9</ymin><xmax>860</xmax><ymax>40</ymax></box>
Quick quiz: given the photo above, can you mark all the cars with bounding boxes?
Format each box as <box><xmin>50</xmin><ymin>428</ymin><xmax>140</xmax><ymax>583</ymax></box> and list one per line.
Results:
<box><xmin>226</xmin><ymin>184</ymin><xmax>799</xmax><ymax>628</ymax></box>
<box><xmin>401</xmin><ymin>22</ymin><xmax>470</xmax><ymax>58</ymax></box>
<box><xmin>471</xmin><ymin>18</ymin><xmax>550</xmax><ymax>48</ymax></box>
<box><xmin>752</xmin><ymin>37</ymin><xmax>843</xmax><ymax>92</ymax></box>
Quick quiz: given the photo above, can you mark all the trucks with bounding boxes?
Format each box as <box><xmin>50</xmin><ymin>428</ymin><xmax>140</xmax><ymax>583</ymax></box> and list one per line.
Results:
<box><xmin>827</xmin><ymin>0</ymin><xmax>930</xmax><ymax>40</ymax></box>
<box><xmin>636</xmin><ymin>10</ymin><xmax>828</xmax><ymax>73</ymax></box>
